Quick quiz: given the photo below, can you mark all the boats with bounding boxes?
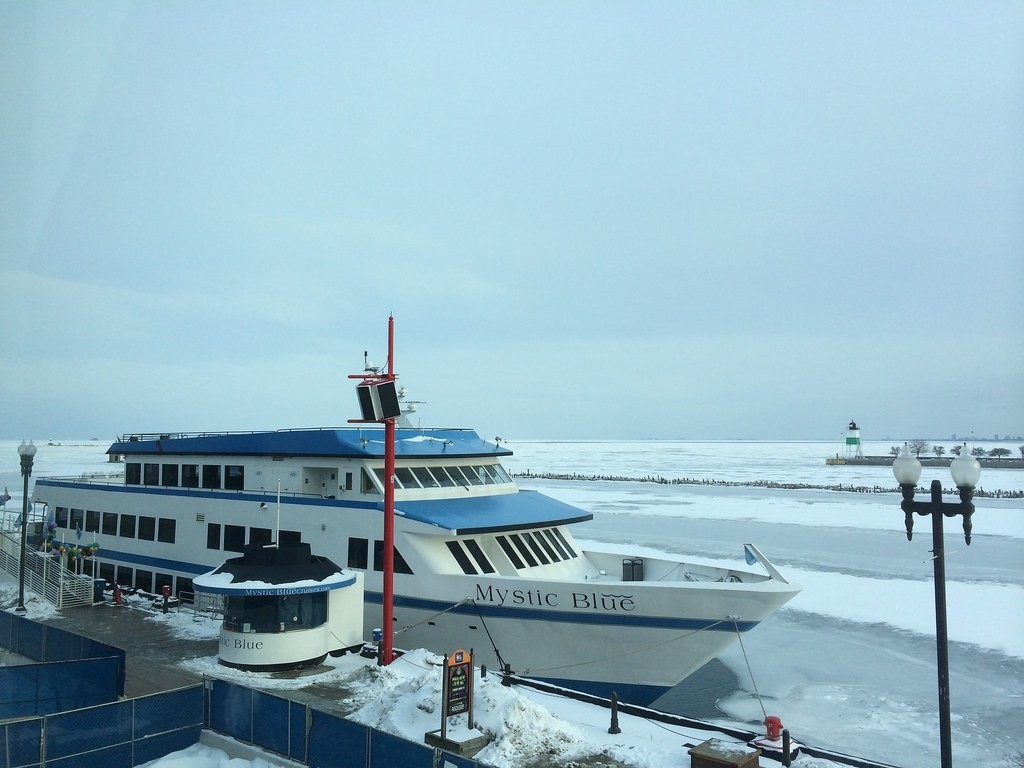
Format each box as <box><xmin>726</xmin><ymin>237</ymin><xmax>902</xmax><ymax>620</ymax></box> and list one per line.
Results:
<box><xmin>13</xmin><ymin>382</ymin><xmax>802</xmax><ymax>709</ymax></box>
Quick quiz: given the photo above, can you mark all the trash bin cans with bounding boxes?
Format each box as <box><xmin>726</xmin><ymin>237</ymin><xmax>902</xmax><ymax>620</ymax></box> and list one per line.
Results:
<box><xmin>93</xmin><ymin>579</ymin><xmax>107</xmax><ymax>602</ymax></box>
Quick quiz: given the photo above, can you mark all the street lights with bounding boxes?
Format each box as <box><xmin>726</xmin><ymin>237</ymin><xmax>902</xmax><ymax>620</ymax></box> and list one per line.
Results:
<box><xmin>14</xmin><ymin>438</ymin><xmax>38</xmax><ymax>614</ymax></box>
<box><xmin>893</xmin><ymin>441</ymin><xmax>982</xmax><ymax>768</ymax></box>
